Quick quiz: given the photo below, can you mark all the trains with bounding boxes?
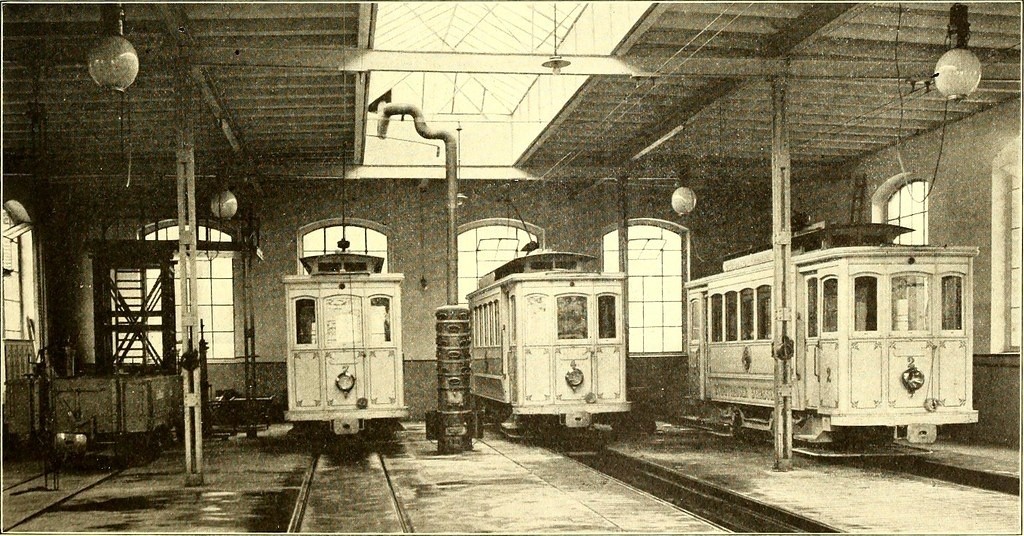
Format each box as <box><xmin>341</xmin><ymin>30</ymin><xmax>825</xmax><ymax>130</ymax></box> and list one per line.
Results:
<box><xmin>281</xmin><ymin>254</ymin><xmax>410</xmax><ymax>434</ymax></box>
<box><xmin>466</xmin><ymin>251</ymin><xmax>633</xmax><ymax>441</ymax></box>
<box><xmin>685</xmin><ymin>221</ymin><xmax>979</xmax><ymax>457</ymax></box>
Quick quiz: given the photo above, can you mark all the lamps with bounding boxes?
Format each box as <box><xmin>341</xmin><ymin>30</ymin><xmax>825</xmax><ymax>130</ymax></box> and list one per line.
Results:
<box><xmin>670</xmin><ymin>171</ymin><xmax>698</xmax><ymax>215</ymax></box>
<box><xmin>934</xmin><ymin>4</ymin><xmax>983</xmax><ymax>101</ymax></box>
<box><xmin>542</xmin><ymin>56</ymin><xmax>572</xmax><ymax>78</ymax></box>
<box><xmin>210</xmin><ymin>167</ymin><xmax>239</xmax><ymax>221</ymax></box>
<box><xmin>86</xmin><ymin>3</ymin><xmax>148</xmax><ymax>96</ymax></box>
